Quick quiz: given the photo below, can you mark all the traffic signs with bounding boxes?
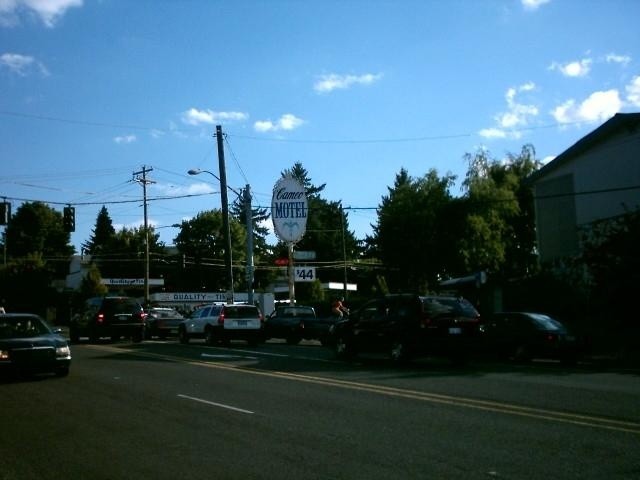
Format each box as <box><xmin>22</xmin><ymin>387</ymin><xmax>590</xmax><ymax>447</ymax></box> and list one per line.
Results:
<box><xmin>294</xmin><ymin>266</ymin><xmax>316</xmax><ymax>282</ymax></box>
<box><xmin>273</xmin><ymin>259</ymin><xmax>290</xmax><ymax>266</ymax></box>
<box><xmin>291</xmin><ymin>250</ymin><xmax>317</xmax><ymax>261</ymax></box>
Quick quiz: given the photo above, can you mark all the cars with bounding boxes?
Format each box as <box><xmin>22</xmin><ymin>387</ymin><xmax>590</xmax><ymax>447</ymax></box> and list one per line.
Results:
<box><xmin>0</xmin><ymin>307</ymin><xmax>6</xmax><ymax>313</ymax></box>
<box><xmin>0</xmin><ymin>312</ymin><xmax>73</xmax><ymax>381</ymax></box>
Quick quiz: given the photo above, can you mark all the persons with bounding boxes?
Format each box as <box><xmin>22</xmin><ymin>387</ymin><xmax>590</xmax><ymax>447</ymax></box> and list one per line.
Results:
<box><xmin>331</xmin><ymin>296</ymin><xmax>350</xmax><ymax>322</ymax></box>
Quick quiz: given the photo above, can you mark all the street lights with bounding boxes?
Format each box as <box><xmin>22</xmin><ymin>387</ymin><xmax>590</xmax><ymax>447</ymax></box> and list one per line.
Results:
<box><xmin>144</xmin><ymin>223</ymin><xmax>183</xmax><ymax>308</ymax></box>
<box><xmin>187</xmin><ymin>169</ymin><xmax>255</xmax><ymax>305</ymax></box>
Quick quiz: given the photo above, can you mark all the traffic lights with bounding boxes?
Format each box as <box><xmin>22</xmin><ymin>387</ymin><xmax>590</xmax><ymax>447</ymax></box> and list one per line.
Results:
<box><xmin>63</xmin><ymin>206</ymin><xmax>76</xmax><ymax>233</ymax></box>
